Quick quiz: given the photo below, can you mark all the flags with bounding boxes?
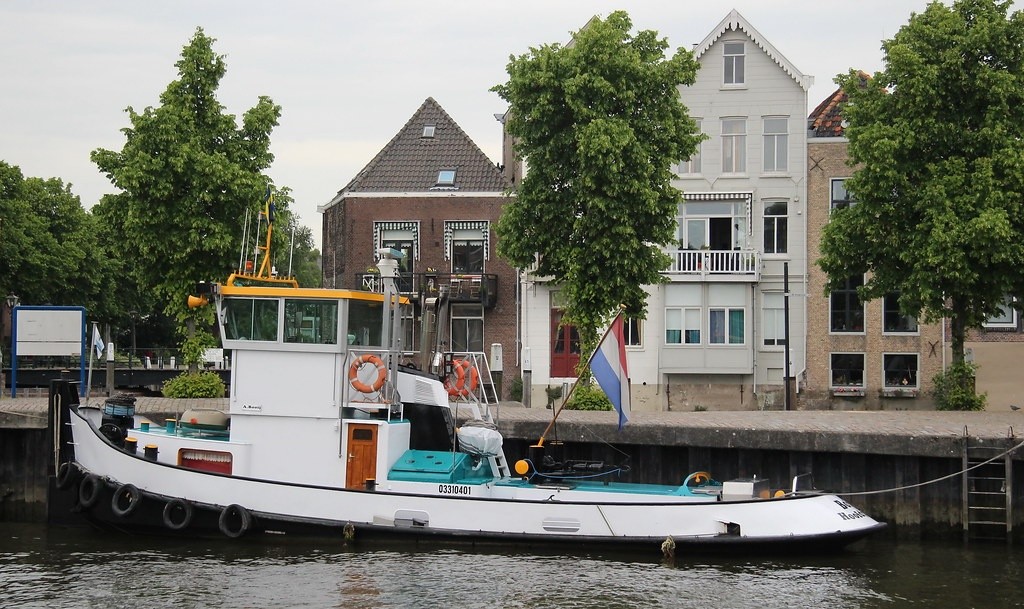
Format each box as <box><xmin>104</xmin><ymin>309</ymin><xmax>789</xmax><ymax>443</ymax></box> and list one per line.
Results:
<box><xmin>94</xmin><ymin>325</ymin><xmax>104</xmax><ymax>360</ymax></box>
<box><xmin>590</xmin><ymin>312</ymin><xmax>630</xmax><ymax>432</ymax></box>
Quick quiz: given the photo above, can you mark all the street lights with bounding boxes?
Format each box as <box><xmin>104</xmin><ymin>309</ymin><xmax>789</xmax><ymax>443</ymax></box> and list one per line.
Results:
<box><xmin>129</xmin><ymin>308</ymin><xmax>138</xmax><ymax>356</ymax></box>
<box><xmin>5</xmin><ymin>291</ymin><xmax>19</xmax><ymax>367</ymax></box>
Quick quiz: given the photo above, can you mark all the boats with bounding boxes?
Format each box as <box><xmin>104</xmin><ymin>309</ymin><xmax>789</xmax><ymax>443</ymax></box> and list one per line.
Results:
<box><xmin>458</xmin><ymin>425</ymin><xmax>503</xmax><ymax>458</ymax></box>
<box><xmin>180</xmin><ymin>408</ymin><xmax>230</xmax><ymax>429</ymax></box>
<box><xmin>47</xmin><ymin>185</ymin><xmax>889</xmax><ymax>556</ymax></box>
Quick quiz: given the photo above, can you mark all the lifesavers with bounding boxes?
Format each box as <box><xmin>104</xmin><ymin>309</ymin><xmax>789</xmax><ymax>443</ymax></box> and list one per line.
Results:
<box><xmin>160</xmin><ymin>498</ymin><xmax>194</xmax><ymax>532</ymax></box>
<box><xmin>441</xmin><ymin>359</ymin><xmax>464</xmax><ymax>395</ymax></box>
<box><xmin>78</xmin><ymin>475</ymin><xmax>102</xmax><ymax>507</ymax></box>
<box><xmin>348</xmin><ymin>355</ymin><xmax>386</xmax><ymax>394</ymax></box>
<box><xmin>447</xmin><ymin>358</ymin><xmax>477</xmax><ymax>395</ymax></box>
<box><xmin>112</xmin><ymin>484</ymin><xmax>141</xmax><ymax>518</ymax></box>
<box><xmin>217</xmin><ymin>504</ymin><xmax>252</xmax><ymax>539</ymax></box>
<box><xmin>55</xmin><ymin>463</ymin><xmax>75</xmax><ymax>491</ymax></box>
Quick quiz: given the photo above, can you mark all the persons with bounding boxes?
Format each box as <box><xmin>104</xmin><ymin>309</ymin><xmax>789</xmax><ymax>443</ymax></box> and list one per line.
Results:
<box><xmin>145</xmin><ymin>349</ymin><xmax>152</xmax><ymax>363</ymax></box>
